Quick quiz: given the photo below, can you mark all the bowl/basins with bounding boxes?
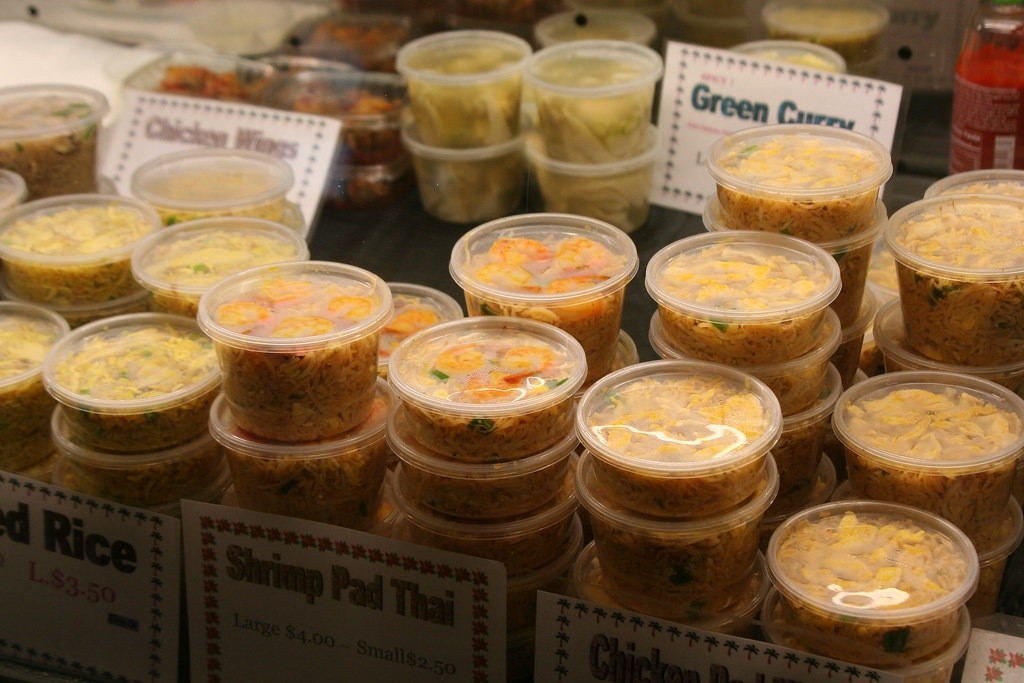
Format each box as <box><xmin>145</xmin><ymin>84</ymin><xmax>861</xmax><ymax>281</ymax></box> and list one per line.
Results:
<box><xmin>0</xmin><ymin>0</ymin><xmax>1024</xmax><ymax>683</ymax></box>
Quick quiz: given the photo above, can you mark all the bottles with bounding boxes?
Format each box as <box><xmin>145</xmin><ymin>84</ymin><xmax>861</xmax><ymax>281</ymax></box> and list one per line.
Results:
<box><xmin>949</xmin><ymin>0</ymin><xmax>1024</xmax><ymax>174</ymax></box>
